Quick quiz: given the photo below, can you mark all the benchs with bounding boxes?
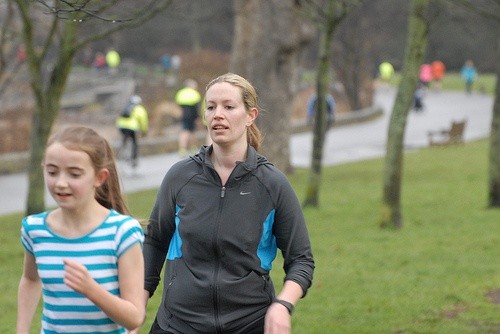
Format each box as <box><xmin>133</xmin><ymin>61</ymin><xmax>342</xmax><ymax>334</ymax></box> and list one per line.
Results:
<box><xmin>428</xmin><ymin>121</ymin><xmax>466</xmax><ymax>146</ymax></box>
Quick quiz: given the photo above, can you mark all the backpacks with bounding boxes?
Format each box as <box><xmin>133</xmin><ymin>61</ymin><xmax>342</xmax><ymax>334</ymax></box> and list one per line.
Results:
<box><xmin>121</xmin><ymin>102</ymin><xmax>136</xmax><ymax>117</ymax></box>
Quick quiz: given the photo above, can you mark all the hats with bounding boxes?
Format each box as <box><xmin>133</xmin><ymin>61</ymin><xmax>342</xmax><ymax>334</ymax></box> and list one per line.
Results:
<box><xmin>131</xmin><ymin>96</ymin><xmax>141</xmax><ymax>104</ymax></box>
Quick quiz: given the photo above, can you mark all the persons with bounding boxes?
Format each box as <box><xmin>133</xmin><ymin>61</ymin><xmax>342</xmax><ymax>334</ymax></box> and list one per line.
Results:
<box><xmin>115</xmin><ymin>96</ymin><xmax>149</xmax><ymax>175</ymax></box>
<box><xmin>14</xmin><ymin>126</ymin><xmax>145</xmax><ymax>334</ymax></box>
<box><xmin>174</xmin><ymin>79</ymin><xmax>201</xmax><ymax>157</ymax></box>
<box><xmin>126</xmin><ymin>72</ymin><xmax>315</xmax><ymax>334</ymax></box>
<box><xmin>306</xmin><ymin>92</ymin><xmax>336</xmax><ymax>140</ymax></box>
<box><xmin>83</xmin><ymin>45</ymin><xmax>121</xmax><ymax>73</ymax></box>
<box><xmin>160</xmin><ymin>51</ymin><xmax>182</xmax><ymax>75</ymax></box>
<box><xmin>380</xmin><ymin>59</ymin><xmax>477</xmax><ymax>109</ymax></box>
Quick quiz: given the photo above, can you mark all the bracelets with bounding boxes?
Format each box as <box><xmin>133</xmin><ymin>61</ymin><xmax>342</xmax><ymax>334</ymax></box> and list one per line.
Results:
<box><xmin>270</xmin><ymin>297</ymin><xmax>295</xmax><ymax>316</ymax></box>
